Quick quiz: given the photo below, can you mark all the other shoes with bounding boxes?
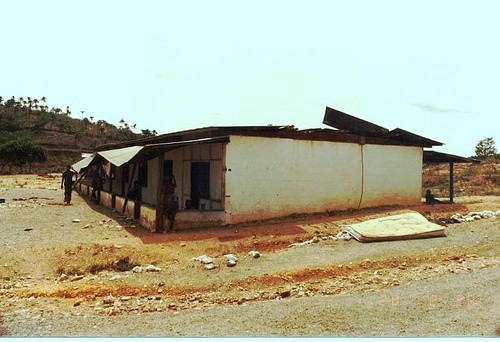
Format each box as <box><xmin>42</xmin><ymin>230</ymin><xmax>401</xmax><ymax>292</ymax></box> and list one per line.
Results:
<box><xmin>157</xmin><ymin>228</ymin><xmax>173</xmax><ymax>233</ymax></box>
<box><xmin>64</xmin><ymin>198</ymin><xmax>70</xmax><ymax>203</ymax></box>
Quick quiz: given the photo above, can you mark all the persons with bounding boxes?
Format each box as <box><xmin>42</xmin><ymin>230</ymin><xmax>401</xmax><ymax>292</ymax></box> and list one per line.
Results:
<box><xmin>425</xmin><ymin>190</ymin><xmax>443</xmax><ymax>204</ymax></box>
<box><xmin>154</xmin><ymin>160</ymin><xmax>178</xmax><ymax>234</ymax></box>
<box><xmin>91</xmin><ymin>172</ymin><xmax>102</xmax><ymax>202</ymax></box>
<box><xmin>184</xmin><ymin>195</ymin><xmax>198</xmax><ymax>210</ymax></box>
<box><xmin>61</xmin><ymin>164</ymin><xmax>79</xmax><ymax>204</ymax></box>
<box><xmin>128</xmin><ymin>181</ymin><xmax>139</xmax><ymax>201</ymax></box>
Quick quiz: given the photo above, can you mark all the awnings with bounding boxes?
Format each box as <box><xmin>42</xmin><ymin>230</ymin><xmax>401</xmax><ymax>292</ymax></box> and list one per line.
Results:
<box><xmin>422</xmin><ymin>149</ymin><xmax>481</xmax><ymax>204</ymax></box>
<box><xmin>71</xmin><ymin>134</ymin><xmax>230</xmax><ymax>174</ymax></box>
